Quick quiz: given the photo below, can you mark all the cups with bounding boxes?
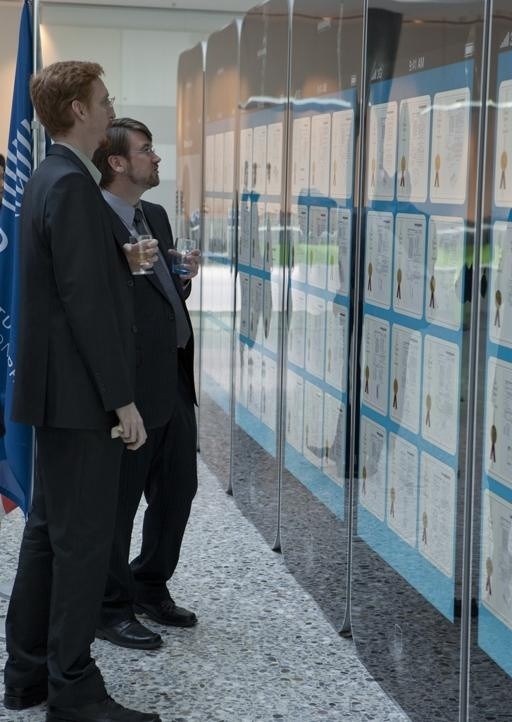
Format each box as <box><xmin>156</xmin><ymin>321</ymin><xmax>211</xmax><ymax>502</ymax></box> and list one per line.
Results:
<box><xmin>172</xmin><ymin>237</ymin><xmax>196</xmax><ymax>275</ymax></box>
<box><xmin>129</xmin><ymin>234</ymin><xmax>154</xmax><ymax>276</ymax></box>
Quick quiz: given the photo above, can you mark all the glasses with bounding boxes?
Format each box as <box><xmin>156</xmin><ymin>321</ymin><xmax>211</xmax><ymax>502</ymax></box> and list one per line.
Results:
<box><xmin>87</xmin><ymin>95</ymin><xmax>116</xmax><ymax>110</ymax></box>
<box><xmin>127</xmin><ymin>147</ymin><xmax>155</xmax><ymax>153</ymax></box>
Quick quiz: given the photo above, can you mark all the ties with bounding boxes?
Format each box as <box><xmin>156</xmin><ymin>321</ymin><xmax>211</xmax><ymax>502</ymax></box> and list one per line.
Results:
<box><xmin>134</xmin><ymin>209</ymin><xmax>192</xmax><ymax>350</ymax></box>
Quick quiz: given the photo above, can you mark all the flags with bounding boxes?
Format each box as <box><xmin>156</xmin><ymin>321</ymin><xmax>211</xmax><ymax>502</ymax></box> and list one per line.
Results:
<box><xmin>1</xmin><ymin>3</ymin><xmax>42</xmax><ymax>515</ymax></box>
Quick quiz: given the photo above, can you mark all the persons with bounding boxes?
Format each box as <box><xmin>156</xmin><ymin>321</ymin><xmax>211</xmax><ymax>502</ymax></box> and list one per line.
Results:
<box><xmin>1</xmin><ymin>58</ymin><xmax>164</xmax><ymax>722</ymax></box>
<box><xmin>90</xmin><ymin>116</ymin><xmax>199</xmax><ymax>652</ymax></box>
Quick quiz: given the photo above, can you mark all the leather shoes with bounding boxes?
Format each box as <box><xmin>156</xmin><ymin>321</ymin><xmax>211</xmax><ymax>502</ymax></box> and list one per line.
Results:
<box><xmin>44</xmin><ymin>692</ymin><xmax>161</xmax><ymax>721</ymax></box>
<box><xmin>94</xmin><ymin>615</ymin><xmax>163</xmax><ymax>651</ymax></box>
<box><xmin>134</xmin><ymin>585</ymin><xmax>197</xmax><ymax>628</ymax></box>
<box><xmin>4</xmin><ymin>685</ymin><xmax>47</xmax><ymax>710</ymax></box>
